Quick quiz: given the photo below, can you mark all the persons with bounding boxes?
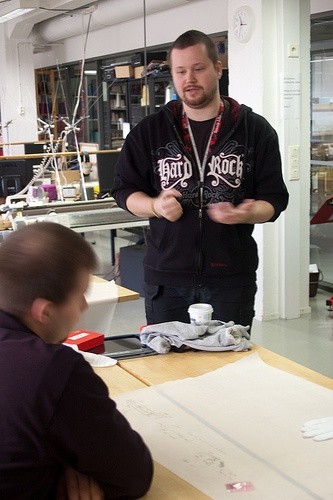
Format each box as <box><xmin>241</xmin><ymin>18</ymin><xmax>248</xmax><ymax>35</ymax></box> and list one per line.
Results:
<box><xmin>0</xmin><ymin>221</ymin><xmax>154</xmax><ymax>500</ymax></box>
<box><xmin>111</xmin><ymin>29</ymin><xmax>289</xmax><ymax>341</ymax></box>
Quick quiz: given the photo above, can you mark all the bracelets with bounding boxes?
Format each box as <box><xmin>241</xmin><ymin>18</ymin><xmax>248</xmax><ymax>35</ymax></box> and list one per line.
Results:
<box><xmin>150</xmin><ymin>199</ymin><xmax>160</xmax><ymax>218</ymax></box>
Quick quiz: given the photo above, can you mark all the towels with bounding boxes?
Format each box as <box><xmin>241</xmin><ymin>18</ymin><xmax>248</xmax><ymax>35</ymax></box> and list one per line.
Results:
<box><xmin>139</xmin><ymin>319</ymin><xmax>254</xmax><ymax>354</ymax></box>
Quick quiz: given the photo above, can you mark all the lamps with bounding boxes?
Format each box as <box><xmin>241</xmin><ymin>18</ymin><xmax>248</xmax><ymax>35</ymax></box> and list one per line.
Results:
<box><xmin>0</xmin><ymin>0</ymin><xmax>40</xmax><ymax>24</ymax></box>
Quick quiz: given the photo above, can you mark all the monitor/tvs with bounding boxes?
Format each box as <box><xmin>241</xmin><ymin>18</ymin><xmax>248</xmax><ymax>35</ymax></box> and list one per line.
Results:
<box><xmin>0</xmin><ymin>142</ymin><xmax>62</xmax><ymax>203</ymax></box>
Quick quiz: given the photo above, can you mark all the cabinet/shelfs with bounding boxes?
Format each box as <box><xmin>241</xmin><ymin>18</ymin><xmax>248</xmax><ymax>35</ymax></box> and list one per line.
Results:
<box><xmin>99</xmin><ymin>77</ymin><xmax>174</xmax><ymax>149</ymax></box>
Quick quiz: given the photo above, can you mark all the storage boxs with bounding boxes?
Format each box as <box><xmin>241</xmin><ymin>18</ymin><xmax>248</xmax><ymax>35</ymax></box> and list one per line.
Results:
<box><xmin>112</xmin><ymin>65</ymin><xmax>145</xmax><ymax>79</ymax></box>
<box><xmin>50</xmin><ymin>170</ymin><xmax>80</xmax><ymax>185</ymax></box>
<box><xmin>64</xmin><ymin>330</ymin><xmax>104</xmax><ymax>351</ymax></box>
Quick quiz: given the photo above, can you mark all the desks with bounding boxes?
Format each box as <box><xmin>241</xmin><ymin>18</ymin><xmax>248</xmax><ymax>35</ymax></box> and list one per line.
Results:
<box><xmin>96</xmin><ymin>343</ymin><xmax>333</xmax><ymax>500</ymax></box>
<box><xmin>84</xmin><ymin>273</ymin><xmax>139</xmax><ymax>306</ymax></box>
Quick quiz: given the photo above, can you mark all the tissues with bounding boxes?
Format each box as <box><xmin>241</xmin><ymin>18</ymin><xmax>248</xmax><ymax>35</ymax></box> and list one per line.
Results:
<box><xmin>39</xmin><ymin>178</ymin><xmax>57</xmax><ymax>200</ymax></box>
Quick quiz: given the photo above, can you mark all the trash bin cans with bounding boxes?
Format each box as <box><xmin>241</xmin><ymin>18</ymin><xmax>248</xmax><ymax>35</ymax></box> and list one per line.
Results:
<box><xmin>309</xmin><ymin>269</ymin><xmax>320</xmax><ymax>297</ymax></box>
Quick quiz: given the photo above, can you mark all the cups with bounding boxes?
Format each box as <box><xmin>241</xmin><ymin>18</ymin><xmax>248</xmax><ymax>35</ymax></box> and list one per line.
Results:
<box><xmin>187</xmin><ymin>303</ymin><xmax>214</xmax><ymax>324</ymax></box>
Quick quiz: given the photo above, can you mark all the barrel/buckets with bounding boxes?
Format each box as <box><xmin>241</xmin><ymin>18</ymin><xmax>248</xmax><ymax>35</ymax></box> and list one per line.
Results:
<box><xmin>309</xmin><ymin>268</ymin><xmax>324</xmax><ymax>297</ymax></box>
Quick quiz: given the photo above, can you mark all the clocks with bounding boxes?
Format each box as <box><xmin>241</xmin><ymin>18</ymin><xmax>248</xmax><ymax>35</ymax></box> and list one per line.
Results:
<box><xmin>231</xmin><ymin>5</ymin><xmax>254</xmax><ymax>44</ymax></box>
